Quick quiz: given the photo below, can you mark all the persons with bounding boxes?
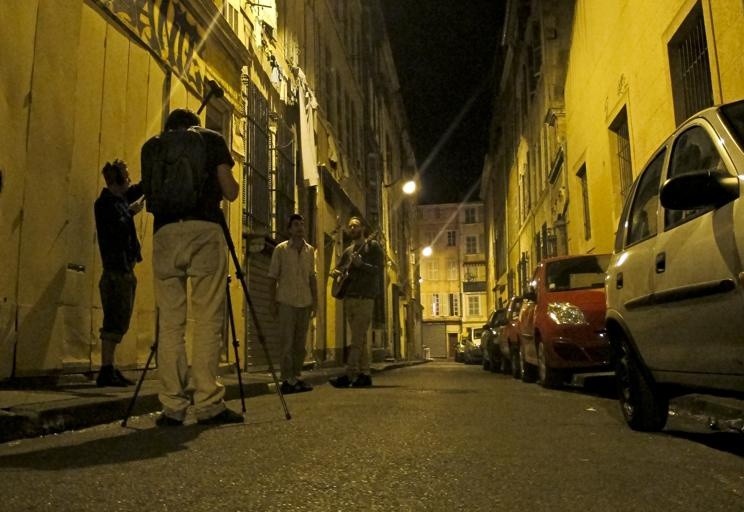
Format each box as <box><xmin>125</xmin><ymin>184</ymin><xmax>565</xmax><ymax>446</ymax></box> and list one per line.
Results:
<box><xmin>268</xmin><ymin>214</ymin><xmax>319</xmax><ymax>394</ymax></box>
<box><xmin>94</xmin><ymin>159</ymin><xmax>143</xmax><ymax>386</ymax></box>
<box><xmin>139</xmin><ymin>108</ymin><xmax>245</xmax><ymax>426</ymax></box>
<box><xmin>329</xmin><ymin>216</ymin><xmax>384</xmax><ymax>388</ymax></box>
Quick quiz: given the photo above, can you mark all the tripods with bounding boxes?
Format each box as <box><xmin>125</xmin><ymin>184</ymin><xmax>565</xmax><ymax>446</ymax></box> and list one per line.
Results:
<box><xmin>119</xmin><ymin>205</ymin><xmax>292</xmax><ymax>428</ymax></box>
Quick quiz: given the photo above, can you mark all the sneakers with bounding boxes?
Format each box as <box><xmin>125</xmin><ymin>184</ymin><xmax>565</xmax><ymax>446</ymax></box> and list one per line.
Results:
<box><xmin>197</xmin><ymin>408</ymin><xmax>244</xmax><ymax>425</ymax></box>
<box><xmin>352</xmin><ymin>373</ymin><xmax>372</xmax><ymax>386</ymax></box>
<box><xmin>96</xmin><ymin>365</ymin><xmax>128</xmax><ymax>388</ymax></box>
<box><xmin>328</xmin><ymin>376</ymin><xmax>351</xmax><ymax>388</ymax></box>
<box><xmin>114</xmin><ymin>368</ymin><xmax>135</xmax><ymax>385</ymax></box>
<box><xmin>281</xmin><ymin>380</ymin><xmax>314</xmax><ymax>394</ymax></box>
<box><xmin>155</xmin><ymin>413</ymin><xmax>184</xmax><ymax>427</ymax></box>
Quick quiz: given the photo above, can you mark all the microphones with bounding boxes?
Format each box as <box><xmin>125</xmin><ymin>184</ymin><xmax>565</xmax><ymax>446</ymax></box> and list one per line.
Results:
<box><xmin>208</xmin><ymin>78</ymin><xmax>226</xmax><ymax>100</ymax></box>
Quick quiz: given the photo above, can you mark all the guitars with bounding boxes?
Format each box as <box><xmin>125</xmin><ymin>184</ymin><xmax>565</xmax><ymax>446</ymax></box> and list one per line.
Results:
<box><xmin>332</xmin><ymin>230</ymin><xmax>379</xmax><ymax>300</ymax></box>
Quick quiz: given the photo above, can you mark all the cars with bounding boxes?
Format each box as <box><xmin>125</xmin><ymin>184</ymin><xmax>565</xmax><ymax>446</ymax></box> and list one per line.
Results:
<box><xmin>603</xmin><ymin>98</ymin><xmax>744</xmax><ymax>433</ymax></box>
<box><xmin>500</xmin><ymin>295</ymin><xmax>523</xmax><ymax>379</ymax></box>
<box><xmin>465</xmin><ymin>326</ymin><xmax>482</xmax><ymax>365</ymax></box>
<box><xmin>455</xmin><ymin>332</ymin><xmax>469</xmax><ymax>363</ymax></box>
<box><xmin>516</xmin><ymin>252</ymin><xmax>611</xmax><ymax>389</ymax></box>
<box><xmin>480</xmin><ymin>308</ymin><xmax>503</xmax><ymax>373</ymax></box>
